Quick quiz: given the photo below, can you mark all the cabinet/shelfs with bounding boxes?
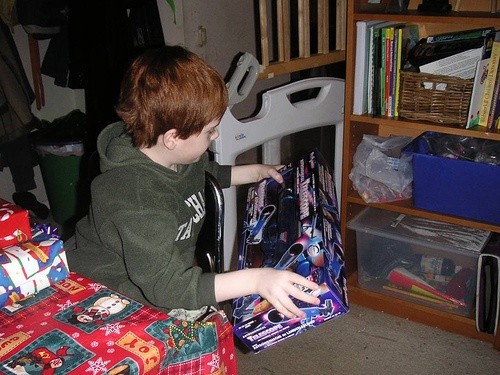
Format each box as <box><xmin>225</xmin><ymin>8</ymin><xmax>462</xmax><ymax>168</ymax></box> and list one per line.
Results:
<box><xmin>340</xmin><ymin>0</ymin><xmax>500</xmax><ymax>352</ymax></box>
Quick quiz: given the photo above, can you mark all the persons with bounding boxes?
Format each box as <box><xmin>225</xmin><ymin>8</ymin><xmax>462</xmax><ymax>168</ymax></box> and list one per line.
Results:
<box><xmin>62</xmin><ymin>44</ymin><xmax>320</xmax><ymax>320</ymax></box>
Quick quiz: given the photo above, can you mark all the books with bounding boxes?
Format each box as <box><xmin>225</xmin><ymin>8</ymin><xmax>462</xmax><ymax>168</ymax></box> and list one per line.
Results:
<box><xmin>356</xmin><ymin>18</ymin><xmax>499</xmax><ymax>129</ymax></box>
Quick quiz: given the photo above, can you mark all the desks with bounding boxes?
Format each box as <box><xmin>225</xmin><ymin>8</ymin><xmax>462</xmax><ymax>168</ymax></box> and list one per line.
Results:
<box><xmin>0</xmin><ymin>272</ymin><xmax>238</xmax><ymax>375</ymax></box>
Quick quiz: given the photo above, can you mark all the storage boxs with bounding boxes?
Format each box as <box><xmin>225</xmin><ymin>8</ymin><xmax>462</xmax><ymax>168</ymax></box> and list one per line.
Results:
<box><xmin>0</xmin><ymin>197</ymin><xmax>33</xmax><ymax>250</ymax></box>
<box><xmin>0</xmin><ymin>227</ymin><xmax>72</xmax><ymax>308</ymax></box>
<box><xmin>234</xmin><ymin>146</ymin><xmax>351</xmax><ymax>354</ymax></box>
<box><xmin>403</xmin><ymin>130</ymin><xmax>500</xmax><ymax>227</ymax></box>
<box><xmin>343</xmin><ymin>205</ymin><xmax>491</xmax><ymax>317</ymax></box>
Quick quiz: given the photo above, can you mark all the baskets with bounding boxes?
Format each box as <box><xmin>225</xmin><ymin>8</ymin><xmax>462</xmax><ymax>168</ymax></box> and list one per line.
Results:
<box><xmin>396</xmin><ymin>68</ymin><xmax>474</xmax><ymax>127</ymax></box>
